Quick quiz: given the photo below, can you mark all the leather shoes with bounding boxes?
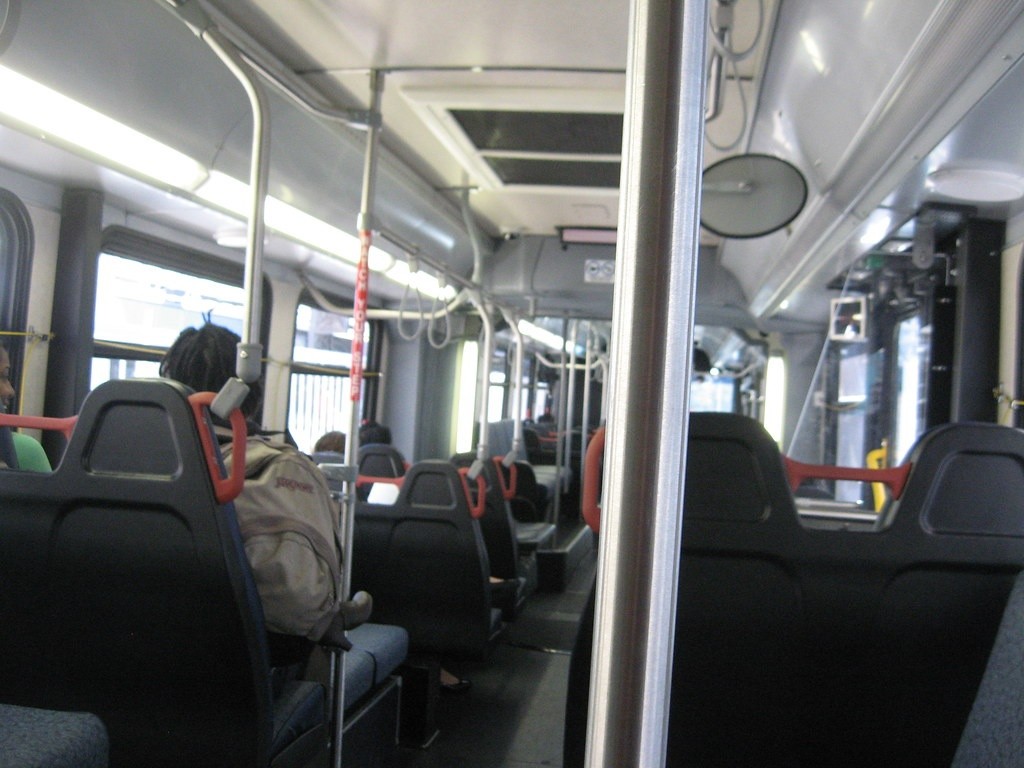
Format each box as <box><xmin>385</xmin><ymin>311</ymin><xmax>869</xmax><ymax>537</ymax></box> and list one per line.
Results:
<box><xmin>443</xmin><ymin>678</ymin><xmax>471</xmax><ymax>693</ymax></box>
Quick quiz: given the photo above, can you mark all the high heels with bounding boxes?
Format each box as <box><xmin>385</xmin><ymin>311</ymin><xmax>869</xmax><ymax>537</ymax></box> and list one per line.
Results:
<box><xmin>322</xmin><ymin>590</ymin><xmax>372</xmax><ymax>652</ymax></box>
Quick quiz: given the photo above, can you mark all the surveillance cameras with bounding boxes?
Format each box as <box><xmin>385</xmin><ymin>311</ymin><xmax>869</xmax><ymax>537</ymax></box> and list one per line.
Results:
<box><xmin>500</xmin><ymin>226</ymin><xmax>519</xmax><ymax>240</ymax></box>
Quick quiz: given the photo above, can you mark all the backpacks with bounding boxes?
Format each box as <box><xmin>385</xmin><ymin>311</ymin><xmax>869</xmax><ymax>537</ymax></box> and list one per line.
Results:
<box><xmin>217</xmin><ymin>438</ymin><xmax>343</xmax><ymax>643</ymax></box>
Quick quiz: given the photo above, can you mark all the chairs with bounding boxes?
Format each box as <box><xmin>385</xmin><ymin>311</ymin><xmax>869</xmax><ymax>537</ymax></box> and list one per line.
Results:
<box><xmin>0</xmin><ymin>380</ymin><xmax>1024</xmax><ymax>768</ymax></box>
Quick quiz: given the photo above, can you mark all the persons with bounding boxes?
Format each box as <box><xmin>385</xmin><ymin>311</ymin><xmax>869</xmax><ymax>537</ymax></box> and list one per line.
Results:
<box><xmin>313</xmin><ymin>423</ymin><xmax>346</xmax><ymax>457</ymax></box>
<box><xmin>159</xmin><ymin>307</ymin><xmax>471</xmax><ymax>707</ymax></box>
<box><xmin>1</xmin><ymin>323</ymin><xmax>103</xmax><ymax>472</ymax></box>
<box><xmin>359</xmin><ymin>419</ymin><xmax>393</xmax><ymax>453</ymax></box>
